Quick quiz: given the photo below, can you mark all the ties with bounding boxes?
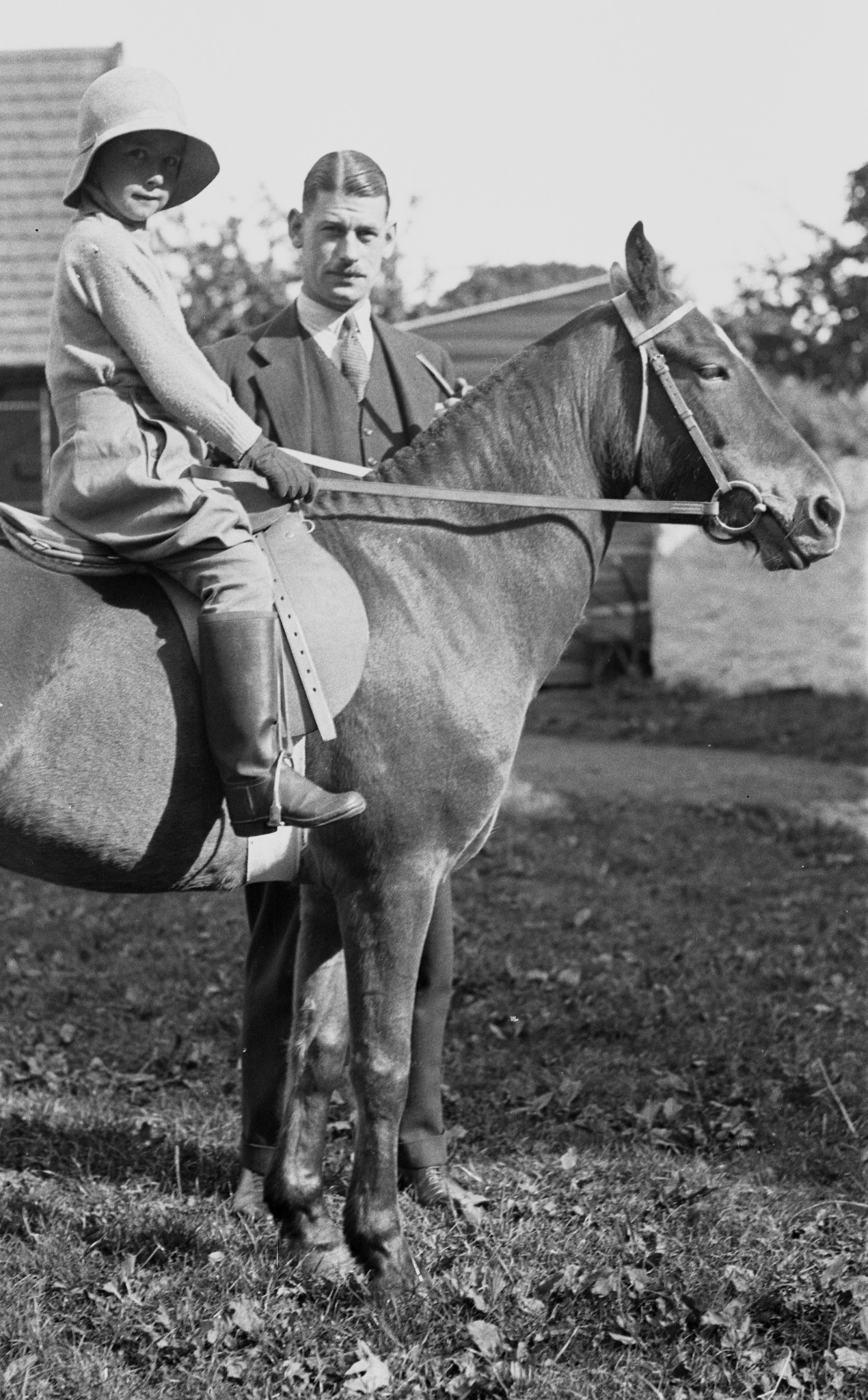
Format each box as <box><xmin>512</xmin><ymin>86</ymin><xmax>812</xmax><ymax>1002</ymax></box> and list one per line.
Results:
<box><xmin>337</xmin><ymin>318</ymin><xmax>367</xmax><ymax>403</ymax></box>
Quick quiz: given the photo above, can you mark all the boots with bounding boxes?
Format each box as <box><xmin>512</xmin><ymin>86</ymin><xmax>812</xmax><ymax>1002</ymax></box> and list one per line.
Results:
<box><xmin>198</xmin><ymin>613</ymin><xmax>369</xmax><ymax>836</ymax></box>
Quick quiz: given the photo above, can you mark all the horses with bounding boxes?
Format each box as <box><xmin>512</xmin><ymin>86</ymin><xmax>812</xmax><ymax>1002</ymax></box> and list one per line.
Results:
<box><xmin>0</xmin><ymin>220</ymin><xmax>846</xmax><ymax>1308</ymax></box>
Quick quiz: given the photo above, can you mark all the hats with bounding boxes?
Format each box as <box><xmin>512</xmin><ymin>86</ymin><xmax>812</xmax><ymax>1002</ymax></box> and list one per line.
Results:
<box><xmin>58</xmin><ymin>66</ymin><xmax>219</xmax><ymax>211</ymax></box>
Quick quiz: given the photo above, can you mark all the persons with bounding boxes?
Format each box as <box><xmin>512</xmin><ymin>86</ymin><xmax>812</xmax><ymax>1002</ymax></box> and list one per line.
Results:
<box><xmin>41</xmin><ymin>67</ymin><xmax>368</xmax><ymax>836</ymax></box>
<box><xmin>201</xmin><ymin>149</ymin><xmax>487</xmax><ymax>1218</ymax></box>
